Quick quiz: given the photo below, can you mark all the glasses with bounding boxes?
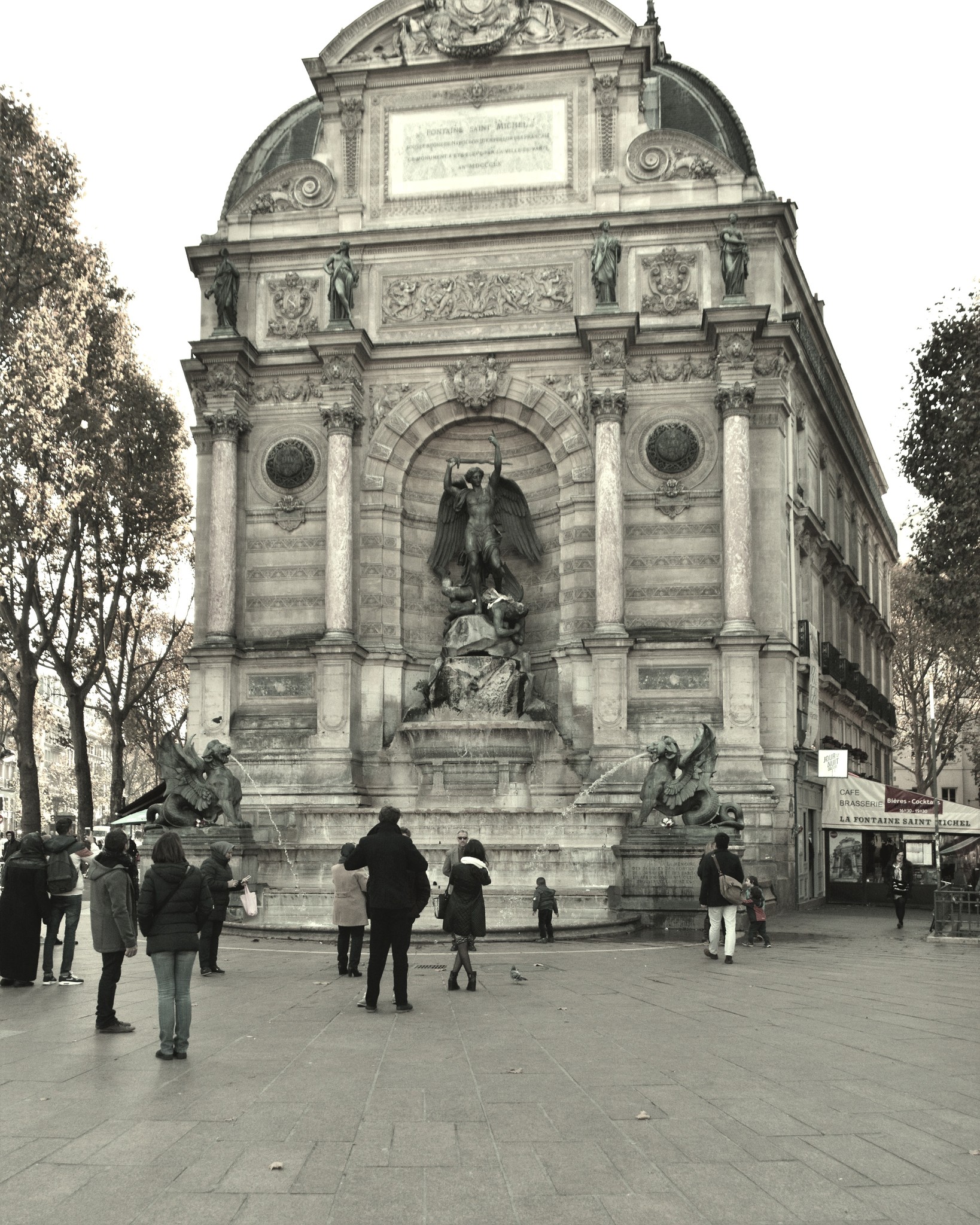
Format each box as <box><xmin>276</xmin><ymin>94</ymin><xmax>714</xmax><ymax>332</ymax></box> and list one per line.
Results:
<box><xmin>897</xmin><ymin>854</ymin><xmax>904</xmax><ymax>857</ymax></box>
<box><xmin>126</xmin><ymin>834</ymin><xmax>132</xmax><ymax>843</ymax></box>
<box><xmin>457</xmin><ymin>836</ymin><xmax>468</xmax><ymax>840</ymax></box>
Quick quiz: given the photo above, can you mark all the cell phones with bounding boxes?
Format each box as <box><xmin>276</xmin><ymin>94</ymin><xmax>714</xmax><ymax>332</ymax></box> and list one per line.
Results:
<box><xmin>85</xmin><ymin>827</ymin><xmax>90</xmax><ymax>838</ymax></box>
<box><xmin>240</xmin><ymin>876</ymin><xmax>251</xmax><ymax>883</ymax></box>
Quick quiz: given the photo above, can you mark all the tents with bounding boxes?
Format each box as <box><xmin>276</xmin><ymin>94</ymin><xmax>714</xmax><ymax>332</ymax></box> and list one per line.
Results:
<box><xmin>821</xmin><ymin>773</ymin><xmax>980</xmax><ymax>835</ymax></box>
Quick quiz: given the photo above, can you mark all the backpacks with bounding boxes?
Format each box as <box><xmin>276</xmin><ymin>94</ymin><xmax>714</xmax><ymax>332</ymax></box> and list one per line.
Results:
<box><xmin>126</xmin><ymin>840</ymin><xmax>137</xmax><ymax>857</ymax></box>
<box><xmin>47</xmin><ymin>849</ymin><xmax>79</xmax><ymax>894</ymax></box>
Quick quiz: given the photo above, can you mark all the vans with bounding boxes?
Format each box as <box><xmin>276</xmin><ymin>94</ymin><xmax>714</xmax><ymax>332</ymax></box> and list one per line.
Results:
<box><xmin>92</xmin><ymin>825</ymin><xmax>111</xmax><ymax>847</ymax></box>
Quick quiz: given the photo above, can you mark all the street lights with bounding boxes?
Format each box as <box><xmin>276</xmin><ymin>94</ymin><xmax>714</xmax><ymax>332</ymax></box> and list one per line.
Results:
<box><xmin>928</xmin><ymin>681</ymin><xmax>944</xmax><ymax>891</ymax></box>
<box><xmin>16</xmin><ymin>814</ymin><xmax>19</xmax><ymax>838</ymax></box>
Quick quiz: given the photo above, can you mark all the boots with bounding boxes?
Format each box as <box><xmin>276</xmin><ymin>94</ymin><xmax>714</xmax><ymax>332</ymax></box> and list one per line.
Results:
<box><xmin>702</xmin><ymin>919</ymin><xmax>711</xmax><ymax>943</ymax></box>
<box><xmin>448</xmin><ymin>971</ymin><xmax>460</xmax><ymax>990</ymax></box>
<box><xmin>466</xmin><ymin>971</ymin><xmax>477</xmax><ymax>991</ymax></box>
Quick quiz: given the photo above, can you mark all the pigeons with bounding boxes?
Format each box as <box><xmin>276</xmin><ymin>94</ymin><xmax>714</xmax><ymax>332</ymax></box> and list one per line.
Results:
<box><xmin>509</xmin><ymin>965</ymin><xmax>528</xmax><ymax>985</ymax></box>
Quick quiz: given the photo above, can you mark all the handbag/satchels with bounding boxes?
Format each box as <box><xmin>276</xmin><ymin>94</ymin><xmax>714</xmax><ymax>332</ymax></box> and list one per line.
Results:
<box><xmin>403</xmin><ymin>836</ymin><xmax>431</xmax><ymax>919</ymax></box>
<box><xmin>139</xmin><ymin>915</ymin><xmax>152</xmax><ymax>937</ymax></box>
<box><xmin>719</xmin><ymin>874</ymin><xmax>743</xmax><ymax>905</ymax></box>
<box><xmin>239</xmin><ymin>883</ymin><xmax>258</xmax><ymax>916</ymax></box>
<box><xmin>433</xmin><ymin>882</ymin><xmax>452</xmax><ymax>919</ymax></box>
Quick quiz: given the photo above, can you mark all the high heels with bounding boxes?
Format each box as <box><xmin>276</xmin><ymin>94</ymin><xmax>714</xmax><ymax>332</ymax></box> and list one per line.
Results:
<box><xmin>338</xmin><ymin>965</ymin><xmax>348</xmax><ymax>975</ymax></box>
<box><xmin>348</xmin><ymin>966</ymin><xmax>362</xmax><ymax>977</ymax></box>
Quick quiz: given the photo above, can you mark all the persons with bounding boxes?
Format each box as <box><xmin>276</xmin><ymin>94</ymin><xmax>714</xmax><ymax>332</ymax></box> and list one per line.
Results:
<box><xmin>739</xmin><ymin>888</ymin><xmax>771</xmax><ymax>948</ymax></box>
<box><xmin>1</xmin><ymin>816</ymin><xmax>136</xmax><ymax>1034</ymax></box>
<box><xmin>699</xmin><ymin>840</ymin><xmax>726</xmax><ymax>946</ymax></box>
<box><xmin>433</xmin><ymin>564</ymin><xmax>529</xmax><ymax>645</ymax></box>
<box><xmin>357</xmin><ymin>826</ymin><xmax>411</xmax><ymax>1007</ymax></box>
<box><xmin>136</xmin><ymin>831</ymin><xmax>213</xmax><ymax>1061</ymax></box>
<box><xmin>744</xmin><ymin>875</ymin><xmax>765</xmax><ymax>942</ymax></box>
<box><xmin>532</xmin><ymin>877</ymin><xmax>559</xmax><ymax>944</ymax></box>
<box><xmin>344</xmin><ymin>805</ymin><xmax>431</xmax><ymax>1013</ymax></box>
<box><xmin>442</xmin><ymin>830</ymin><xmax>477</xmax><ymax>951</ymax></box>
<box><xmin>883</xmin><ymin>850</ymin><xmax>914</xmax><ymax>928</ymax></box>
<box><xmin>697</xmin><ymin>832</ymin><xmax>745</xmax><ymax>964</ymax></box>
<box><xmin>198</xmin><ymin>841</ymin><xmax>250</xmax><ymax>976</ymax></box>
<box><xmin>331</xmin><ymin>842</ymin><xmax>369</xmax><ymax>978</ymax></box>
<box><xmin>442</xmin><ymin>837</ymin><xmax>491</xmax><ymax>992</ymax></box>
<box><xmin>444</xmin><ymin>435</ymin><xmax>504</xmax><ymax>615</ymax></box>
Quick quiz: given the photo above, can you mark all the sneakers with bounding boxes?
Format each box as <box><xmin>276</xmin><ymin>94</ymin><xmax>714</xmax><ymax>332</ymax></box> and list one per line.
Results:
<box><xmin>365</xmin><ymin>1004</ymin><xmax>377</xmax><ymax>1012</ymax></box>
<box><xmin>59</xmin><ymin>973</ymin><xmax>84</xmax><ymax>985</ymax></box>
<box><xmin>396</xmin><ymin>1004</ymin><xmax>413</xmax><ymax>1013</ymax></box>
<box><xmin>200</xmin><ymin>967</ymin><xmax>212</xmax><ymax>976</ymax></box>
<box><xmin>742</xmin><ymin>941</ymin><xmax>753</xmax><ymax>947</ymax></box>
<box><xmin>210</xmin><ymin>966</ymin><xmax>225</xmax><ymax>974</ymax></box>
<box><xmin>156</xmin><ymin>1049</ymin><xmax>173</xmax><ymax>1060</ymax></box>
<box><xmin>392</xmin><ymin>992</ymin><xmax>397</xmax><ymax>1003</ymax></box>
<box><xmin>753</xmin><ymin>934</ymin><xmax>763</xmax><ymax>941</ymax></box>
<box><xmin>174</xmin><ymin>1048</ymin><xmax>187</xmax><ymax>1059</ymax></box>
<box><xmin>96</xmin><ymin>1019</ymin><xmax>135</xmax><ymax>1033</ymax></box>
<box><xmin>762</xmin><ymin>942</ymin><xmax>771</xmax><ymax>947</ymax></box>
<box><xmin>357</xmin><ymin>993</ymin><xmax>367</xmax><ymax>1006</ymax></box>
<box><xmin>42</xmin><ymin>975</ymin><xmax>57</xmax><ymax>985</ymax></box>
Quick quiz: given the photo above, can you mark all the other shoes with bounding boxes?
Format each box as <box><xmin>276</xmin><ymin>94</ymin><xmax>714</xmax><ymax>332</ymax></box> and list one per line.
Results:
<box><xmin>13</xmin><ymin>980</ymin><xmax>34</xmax><ymax>987</ymax></box>
<box><xmin>39</xmin><ymin>936</ymin><xmax>46</xmax><ymax>944</ymax></box>
<box><xmin>0</xmin><ymin>978</ymin><xmax>15</xmax><ymax>985</ymax></box>
<box><xmin>897</xmin><ymin>923</ymin><xmax>904</xmax><ymax>928</ymax></box>
<box><xmin>74</xmin><ymin>941</ymin><xmax>78</xmax><ymax>945</ymax></box>
<box><xmin>547</xmin><ymin>937</ymin><xmax>554</xmax><ymax>943</ymax></box>
<box><xmin>704</xmin><ymin>948</ymin><xmax>719</xmax><ymax>960</ymax></box>
<box><xmin>720</xmin><ymin>940</ymin><xmax>725</xmax><ymax>945</ymax></box>
<box><xmin>54</xmin><ymin>937</ymin><xmax>62</xmax><ymax>945</ymax></box>
<box><xmin>704</xmin><ymin>941</ymin><xmax>711</xmax><ymax>945</ymax></box>
<box><xmin>724</xmin><ymin>955</ymin><xmax>733</xmax><ymax>964</ymax></box>
<box><xmin>535</xmin><ymin>938</ymin><xmax>547</xmax><ymax>943</ymax></box>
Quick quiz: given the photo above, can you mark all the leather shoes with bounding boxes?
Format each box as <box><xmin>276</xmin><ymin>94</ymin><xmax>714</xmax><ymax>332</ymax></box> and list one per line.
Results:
<box><xmin>451</xmin><ymin>940</ymin><xmax>459</xmax><ymax>951</ymax></box>
<box><xmin>467</xmin><ymin>941</ymin><xmax>476</xmax><ymax>951</ymax></box>
